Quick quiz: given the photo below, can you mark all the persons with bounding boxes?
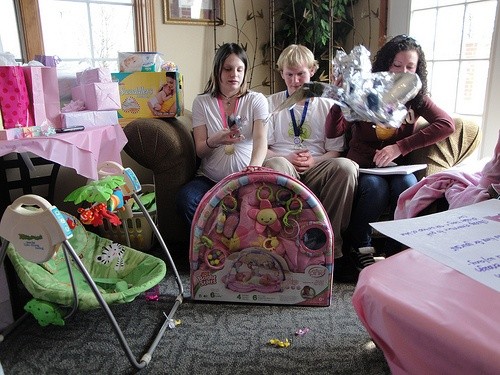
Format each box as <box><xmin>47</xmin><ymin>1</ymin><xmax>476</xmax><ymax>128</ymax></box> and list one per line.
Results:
<box><xmin>263</xmin><ymin>43</ymin><xmax>360</xmax><ymax>284</ymax></box>
<box><xmin>326</xmin><ymin>33</ymin><xmax>455</xmax><ymax>268</ymax></box>
<box><xmin>147</xmin><ymin>72</ymin><xmax>177</xmax><ymax>117</ymax></box>
<box><xmin>181</xmin><ymin>42</ymin><xmax>270</xmax><ymax>228</ymax></box>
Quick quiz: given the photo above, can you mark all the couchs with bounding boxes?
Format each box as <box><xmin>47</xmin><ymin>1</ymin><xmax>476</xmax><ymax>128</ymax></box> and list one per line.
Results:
<box><xmin>122</xmin><ymin>113</ymin><xmax>479</xmax><ymax>268</ymax></box>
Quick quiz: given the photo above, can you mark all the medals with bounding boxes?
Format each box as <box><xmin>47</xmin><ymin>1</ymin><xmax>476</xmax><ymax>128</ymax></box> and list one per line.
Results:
<box><xmin>294</xmin><ymin>137</ymin><xmax>300</xmax><ymax>144</ymax></box>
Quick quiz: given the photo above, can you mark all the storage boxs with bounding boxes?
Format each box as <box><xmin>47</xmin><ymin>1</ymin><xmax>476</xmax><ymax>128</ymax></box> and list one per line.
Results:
<box><xmin>117</xmin><ymin>52</ymin><xmax>164</xmax><ymax>73</ymax></box>
<box><xmin>75</xmin><ymin>67</ymin><xmax>113</xmax><ymax>85</ymax></box>
<box><xmin>72</xmin><ymin>83</ymin><xmax>121</xmax><ymax>111</ymax></box>
<box><xmin>59</xmin><ymin>110</ymin><xmax>118</xmax><ymax>129</ymax></box>
<box><xmin>101</xmin><ymin>183</ymin><xmax>156</xmax><ymax>250</ymax></box>
<box><xmin>111</xmin><ymin>72</ymin><xmax>184</xmax><ymax>129</ymax></box>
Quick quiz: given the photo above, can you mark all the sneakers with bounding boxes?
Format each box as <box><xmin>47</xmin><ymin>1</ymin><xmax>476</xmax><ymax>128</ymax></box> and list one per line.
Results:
<box><xmin>342</xmin><ymin>244</ymin><xmax>376</xmax><ymax>271</ymax></box>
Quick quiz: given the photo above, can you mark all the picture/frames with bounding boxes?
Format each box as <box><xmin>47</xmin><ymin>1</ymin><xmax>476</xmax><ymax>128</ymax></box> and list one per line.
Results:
<box><xmin>163</xmin><ymin>0</ymin><xmax>226</xmax><ymax>27</ymax></box>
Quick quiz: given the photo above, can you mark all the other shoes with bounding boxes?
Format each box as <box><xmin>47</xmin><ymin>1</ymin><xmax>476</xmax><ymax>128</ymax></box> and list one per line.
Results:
<box><xmin>333</xmin><ymin>256</ymin><xmax>359</xmax><ymax>282</ymax></box>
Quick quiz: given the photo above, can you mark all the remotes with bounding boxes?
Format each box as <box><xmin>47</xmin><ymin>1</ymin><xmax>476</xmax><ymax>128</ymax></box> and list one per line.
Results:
<box><xmin>56</xmin><ymin>125</ymin><xmax>84</xmax><ymax>133</ymax></box>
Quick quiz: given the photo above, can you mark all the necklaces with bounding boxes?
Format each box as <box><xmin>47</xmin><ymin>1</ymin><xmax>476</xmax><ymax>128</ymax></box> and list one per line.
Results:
<box><xmin>221</xmin><ymin>92</ymin><xmax>238</xmax><ymax>105</ymax></box>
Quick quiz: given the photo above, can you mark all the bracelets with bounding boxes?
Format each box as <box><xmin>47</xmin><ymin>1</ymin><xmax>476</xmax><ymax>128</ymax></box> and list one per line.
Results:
<box><xmin>206</xmin><ymin>137</ymin><xmax>219</xmax><ymax>148</ymax></box>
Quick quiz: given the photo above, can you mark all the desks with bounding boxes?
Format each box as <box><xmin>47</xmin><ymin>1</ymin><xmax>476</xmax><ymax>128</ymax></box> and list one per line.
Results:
<box><xmin>0</xmin><ymin>123</ymin><xmax>127</xmax><ymax>215</ymax></box>
